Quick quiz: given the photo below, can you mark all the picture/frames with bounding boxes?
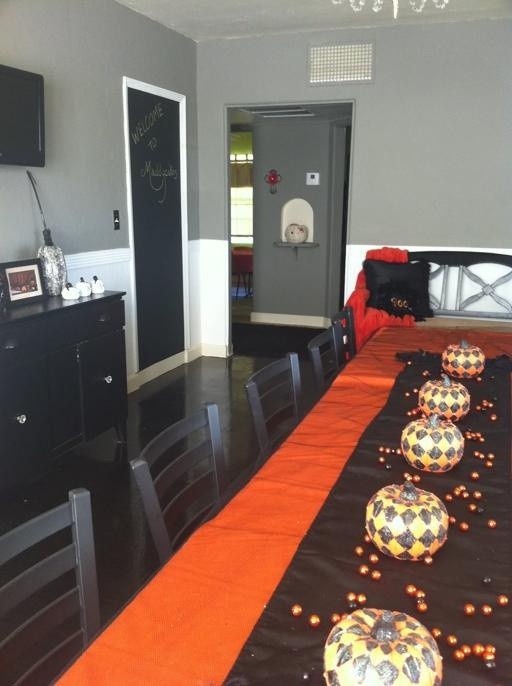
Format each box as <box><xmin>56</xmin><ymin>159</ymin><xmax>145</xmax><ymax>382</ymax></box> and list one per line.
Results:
<box><xmin>2</xmin><ymin>259</ymin><xmax>44</xmax><ymax>304</ymax></box>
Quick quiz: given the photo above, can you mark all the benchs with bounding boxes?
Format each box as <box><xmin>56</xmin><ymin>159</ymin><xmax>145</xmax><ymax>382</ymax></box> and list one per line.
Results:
<box><xmin>404</xmin><ymin>250</ymin><xmax>512</xmax><ymax>317</ymax></box>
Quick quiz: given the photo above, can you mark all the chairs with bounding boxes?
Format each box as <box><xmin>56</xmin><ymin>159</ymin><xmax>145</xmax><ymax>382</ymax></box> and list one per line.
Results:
<box><xmin>308</xmin><ymin>323</ymin><xmax>344</xmax><ymax>398</ymax></box>
<box><xmin>129</xmin><ymin>401</ymin><xmax>231</xmax><ymax>564</ymax></box>
<box><xmin>245</xmin><ymin>352</ymin><xmax>304</xmax><ymax>463</ymax></box>
<box><xmin>2</xmin><ymin>485</ymin><xmax>103</xmax><ymax>685</ymax></box>
<box><xmin>329</xmin><ymin>307</ymin><xmax>356</xmax><ymax>367</ymax></box>
<box><xmin>232</xmin><ymin>247</ymin><xmax>253</xmax><ymax>301</ymax></box>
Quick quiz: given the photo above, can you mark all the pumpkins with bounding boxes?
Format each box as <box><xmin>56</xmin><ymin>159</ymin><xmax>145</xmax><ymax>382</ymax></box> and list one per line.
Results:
<box><xmin>401</xmin><ymin>414</ymin><xmax>464</xmax><ymax>473</ymax></box>
<box><xmin>442</xmin><ymin>340</ymin><xmax>485</xmax><ymax>379</ymax></box>
<box><xmin>366</xmin><ymin>481</ymin><xmax>450</xmax><ymax>561</ymax></box>
<box><xmin>418</xmin><ymin>374</ymin><xmax>470</xmax><ymax>422</ymax></box>
<box><xmin>323</xmin><ymin>608</ymin><xmax>442</xmax><ymax>686</ymax></box>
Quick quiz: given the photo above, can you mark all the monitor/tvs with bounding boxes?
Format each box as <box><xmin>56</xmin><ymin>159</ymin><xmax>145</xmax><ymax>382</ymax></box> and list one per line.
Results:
<box><xmin>0</xmin><ymin>64</ymin><xmax>45</xmax><ymax>168</ymax></box>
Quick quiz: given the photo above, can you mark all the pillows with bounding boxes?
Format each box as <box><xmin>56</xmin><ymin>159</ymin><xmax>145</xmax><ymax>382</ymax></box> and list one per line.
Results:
<box><xmin>362</xmin><ymin>259</ymin><xmax>433</xmax><ymax>319</ymax></box>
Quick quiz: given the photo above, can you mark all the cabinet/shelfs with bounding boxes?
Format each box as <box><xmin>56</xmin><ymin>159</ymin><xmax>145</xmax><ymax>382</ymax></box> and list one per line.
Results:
<box><xmin>2</xmin><ymin>295</ymin><xmax>131</xmax><ymax>483</ymax></box>
